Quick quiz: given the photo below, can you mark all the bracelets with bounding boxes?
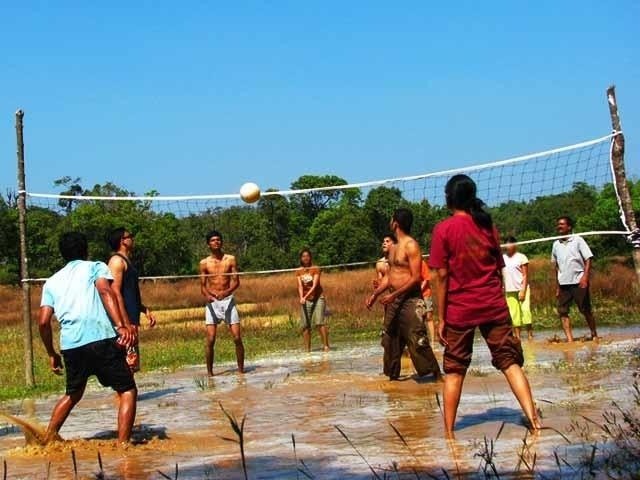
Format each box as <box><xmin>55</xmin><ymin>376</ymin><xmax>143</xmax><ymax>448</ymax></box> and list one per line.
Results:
<box><xmin>117</xmin><ymin>326</ymin><xmax>127</xmax><ymax>330</ymax></box>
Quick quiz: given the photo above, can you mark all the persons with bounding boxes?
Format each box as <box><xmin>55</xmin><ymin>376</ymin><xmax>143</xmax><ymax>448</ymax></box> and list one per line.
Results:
<box><xmin>427</xmin><ymin>174</ymin><xmax>542</xmax><ymax>440</ymax></box>
<box><xmin>106</xmin><ymin>226</ymin><xmax>156</xmax><ymax>432</ymax></box>
<box><xmin>38</xmin><ymin>231</ymin><xmax>136</xmax><ymax>441</ymax></box>
<box><xmin>365</xmin><ymin>207</ymin><xmax>442</xmax><ymax>384</ymax></box>
<box><xmin>551</xmin><ymin>215</ymin><xmax>599</xmax><ymax>342</ymax></box>
<box><xmin>501</xmin><ymin>235</ymin><xmax>533</xmax><ymax>338</ymax></box>
<box><xmin>295</xmin><ymin>249</ymin><xmax>330</xmax><ymax>352</ymax></box>
<box><xmin>198</xmin><ymin>231</ymin><xmax>245</xmax><ymax>376</ymax></box>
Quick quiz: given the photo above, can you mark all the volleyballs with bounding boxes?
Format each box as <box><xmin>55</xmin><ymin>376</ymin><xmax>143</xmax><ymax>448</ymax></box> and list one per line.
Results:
<box><xmin>240</xmin><ymin>182</ymin><xmax>260</xmax><ymax>203</ymax></box>
